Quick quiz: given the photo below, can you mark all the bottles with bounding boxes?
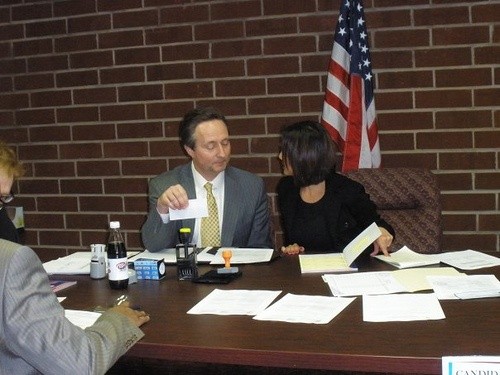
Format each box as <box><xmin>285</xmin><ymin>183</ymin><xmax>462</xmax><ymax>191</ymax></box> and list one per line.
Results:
<box><xmin>106</xmin><ymin>221</ymin><xmax>129</xmax><ymax>290</ymax></box>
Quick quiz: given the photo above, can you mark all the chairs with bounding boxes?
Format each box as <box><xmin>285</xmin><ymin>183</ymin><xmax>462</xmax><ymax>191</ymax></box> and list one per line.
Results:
<box><xmin>346</xmin><ymin>168</ymin><xmax>443</xmax><ymax>255</ymax></box>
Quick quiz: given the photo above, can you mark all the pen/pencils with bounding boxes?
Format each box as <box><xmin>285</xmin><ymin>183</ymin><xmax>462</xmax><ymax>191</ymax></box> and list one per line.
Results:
<box><xmin>272</xmin><ymin>252</ymin><xmax>287</xmax><ymax>262</ymax></box>
<box><xmin>107</xmin><ymin>304</ymin><xmax>143</xmax><ymax>310</ymax></box>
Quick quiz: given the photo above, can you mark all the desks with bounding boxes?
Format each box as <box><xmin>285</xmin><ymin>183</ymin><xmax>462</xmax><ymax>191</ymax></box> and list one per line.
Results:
<box><xmin>38</xmin><ymin>251</ymin><xmax>500</xmax><ymax>375</ymax></box>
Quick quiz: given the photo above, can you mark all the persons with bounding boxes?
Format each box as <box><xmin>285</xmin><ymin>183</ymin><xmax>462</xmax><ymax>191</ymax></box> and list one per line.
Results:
<box><xmin>0</xmin><ymin>142</ymin><xmax>150</xmax><ymax>375</ymax></box>
<box><xmin>142</xmin><ymin>108</ymin><xmax>273</xmax><ymax>249</ymax></box>
<box><xmin>276</xmin><ymin>121</ymin><xmax>395</xmax><ymax>257</ymax></box>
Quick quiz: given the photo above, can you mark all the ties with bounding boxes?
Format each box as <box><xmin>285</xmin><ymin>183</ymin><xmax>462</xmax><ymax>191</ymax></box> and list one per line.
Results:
<box><xmin>201</xmin><ymin>183</ymin><xmax>221</xmax><ymax>248</ymax></box>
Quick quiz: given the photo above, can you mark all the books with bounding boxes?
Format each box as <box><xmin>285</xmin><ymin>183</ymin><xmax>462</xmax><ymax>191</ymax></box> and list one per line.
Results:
<box><xmin>299</xmin><ymin>222</ymin><xmax>382</xmax><ymax>274</ymax></box>
<box><xmin>374</xmin><ymin>246</ymin><xmax>440</xmax><ymax>269</ymax></box>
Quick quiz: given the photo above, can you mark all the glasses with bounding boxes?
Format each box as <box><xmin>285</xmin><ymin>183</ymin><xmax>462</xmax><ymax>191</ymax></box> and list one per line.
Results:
<box><xmin>0</xmin><ymin>190</ymin><xmax>15</xmax><ymax>205</ymax></box>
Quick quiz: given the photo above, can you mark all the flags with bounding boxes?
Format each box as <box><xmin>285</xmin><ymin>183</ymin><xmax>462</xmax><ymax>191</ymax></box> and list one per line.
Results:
<box><xmin>320</xmin><ymin>0</ymin><xmax>381</xmax><ymax>172</ymax></box>
